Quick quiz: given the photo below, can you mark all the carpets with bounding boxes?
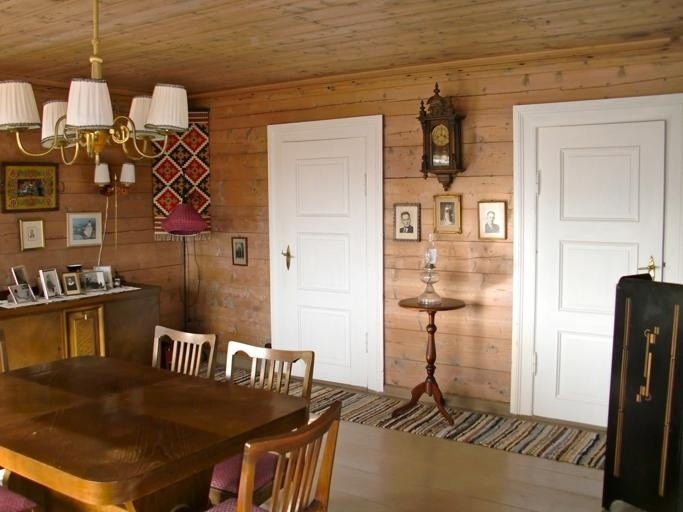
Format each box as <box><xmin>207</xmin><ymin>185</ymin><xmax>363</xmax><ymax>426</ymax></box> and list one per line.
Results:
<box><xmin>162</xmin><ymin>360</ymin><xmax>608</xmax><ymax>471</ymax></box>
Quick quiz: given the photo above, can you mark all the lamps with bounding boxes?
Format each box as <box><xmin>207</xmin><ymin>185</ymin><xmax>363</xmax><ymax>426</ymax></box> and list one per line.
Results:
<box><xmin>94</xmin><ymin>162</ymin><xmax>136</xmax><ymax>196</ymax></box>
<box><xmin>0</xmin><ymin>0</ymin><xmax>190</xmax><ymax>166</ymax></box>
<box><xmin>161</xmin><ymin>169</ymin><xmax>208</xmax><ymax>362</ymax></box>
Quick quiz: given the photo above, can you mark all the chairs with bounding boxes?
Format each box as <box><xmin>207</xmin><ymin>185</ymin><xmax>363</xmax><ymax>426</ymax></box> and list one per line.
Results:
<box><xmin>151</xmin><ymin>325</ymin><xmax>217</xmax><ymax>380</ymax></box>
<box><xmin>208</xmin><ymin>340</ymin><xmax>314</xmax><ymax>505</ymax></box>
<box><xmin>207</xmin><ymin>398</ymin><xmax>341</xmax><ymax>512</ymax></box>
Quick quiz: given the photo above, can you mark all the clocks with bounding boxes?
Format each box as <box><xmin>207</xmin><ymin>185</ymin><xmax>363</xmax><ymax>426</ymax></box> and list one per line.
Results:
<box><xmin>416</xmin><ymin>81</ymin><xmax>466</xmax><ymax>191</ymax></box>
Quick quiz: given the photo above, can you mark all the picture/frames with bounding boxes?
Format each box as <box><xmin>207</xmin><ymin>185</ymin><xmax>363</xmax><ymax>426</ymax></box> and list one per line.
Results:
<box><xmin>477</xmin><ymin>200</ymin><xmax>506</xmax><ymax>240</ymax></box>
<box><xmin>83</xmin><ymin>271</ymin><xmax>107</xmax><ymax>291</ymax></box>
<box><xmin>93</xmin><ymin>265</ymin><xmax>113</xmax><ymax>289</ymax></box>
<box><xmin>39</xmin><ymin>268</ymin><xmax>61</xmax><ymax>300</ymax></box>
<box><xmin>19</xmin><ymin>219</ymin><xmax>46</xmax><ymax>252</ymax></box>
<box><xmin>393</xmin><ymin>203</ymin><xmax>421</xmax><ymax>242</ymax></box>
<box><xmin>231</xmin><ymin>237</ymin><xmax>248</xmax><ymax>266</ymax></box>
<box><xmin>61</xmin><ymin>272</ymin><xmax>80</xmax><ymax>295</ymax></box>
<box><xmin>11</xmin><ymin>265</ymin><xmax>29</xmax><ymax>285</ymax></box>
<box><xmin>0</xmin><ymin>162</ymin><xmax>60</xmax><ymax>213</ymax></box>
<box><xmin>433</xmin><ymin>195</ymin><xmax>462</xmax><ymax>234</ymax></box>
<box><xmin>65</xmin><ymin>211</ymin><xmax>103</xmax><ymax>249</ymax></box>
<box><xmin>8</xmin><ymin>284</ymin><xmax>36</xmax><ymax>304</ymax></box>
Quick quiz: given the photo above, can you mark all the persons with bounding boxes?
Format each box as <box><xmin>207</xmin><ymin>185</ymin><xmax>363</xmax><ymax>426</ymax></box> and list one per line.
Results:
<box><xmin>66</xmin><ymin>277</ymin><xmax>76</xmax><ymax>289</ymax></box>
<box><xmin>46</xmin><ymin>274</ymin><xmax>56</xmax><ymax>293</ymax></box>
<box><xmin>485</xmin><ymin>211</ymin><xmax>499</xmax><ymax>233</ymax></box>
<box><xmin>400</xmin><ymin>212</ymin><xmax>413</xmax><ymax>233</ymax></box>
<box><xmin>440</xmin><ymin>202</ymin><xmax>454</xmax><ymax>225</ymax></box>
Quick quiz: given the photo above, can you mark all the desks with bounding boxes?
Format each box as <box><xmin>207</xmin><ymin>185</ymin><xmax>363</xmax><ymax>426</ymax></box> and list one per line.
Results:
<box><xmin>0</xmin><ymin>356</ymin><xmax>309</xmax><ymax>512</ymax></box>
<box><xmin>392</xmin><ymin>297</ymin><xmax>466</xmax><ymax>425</ymax></box>
<box><xmin>0</xmin><ymin>281</ymin><xmax>161</xmax><ymax>373</ymax></box>
